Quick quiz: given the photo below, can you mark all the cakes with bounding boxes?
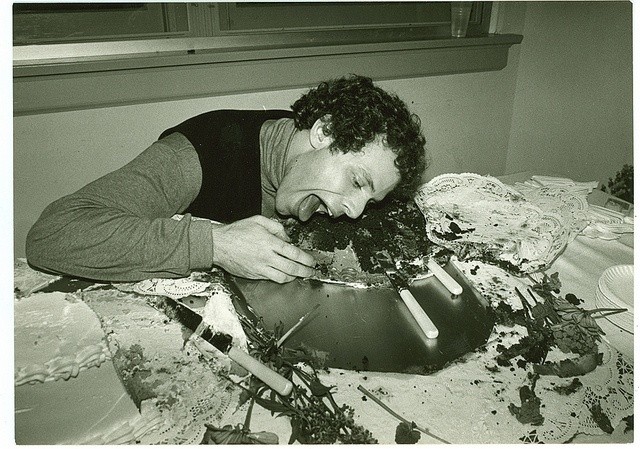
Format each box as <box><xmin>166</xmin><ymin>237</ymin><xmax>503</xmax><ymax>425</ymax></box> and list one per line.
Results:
<box><xmin>278</xmin><ymin>178</ymin><xmax>432</xmax><ymax>289</ymax></box>
<box><xmin>14</xmin><ymin>290</ymin><xmax>163</xmax><ymax>444</ymax></box>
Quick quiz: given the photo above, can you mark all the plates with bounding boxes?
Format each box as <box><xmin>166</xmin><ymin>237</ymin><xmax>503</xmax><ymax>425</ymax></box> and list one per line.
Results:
<box><xmin>595</xmin><ymin>263</ymin><xmax>634</xmax><ymax>334</ymax></box>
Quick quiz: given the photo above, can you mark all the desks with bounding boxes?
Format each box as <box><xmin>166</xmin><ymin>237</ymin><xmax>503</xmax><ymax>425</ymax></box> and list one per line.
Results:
<box><xmin>14</xmin><ymin>168</ymin><xmax>636</xmax><ymax>443</ymax></box>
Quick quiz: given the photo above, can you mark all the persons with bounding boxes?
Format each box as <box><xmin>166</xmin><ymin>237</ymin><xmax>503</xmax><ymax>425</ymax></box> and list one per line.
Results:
<box><xmin>25</xmin><ymin>73</ymin><xmax>427</xmax><ymax>285</ymax></box>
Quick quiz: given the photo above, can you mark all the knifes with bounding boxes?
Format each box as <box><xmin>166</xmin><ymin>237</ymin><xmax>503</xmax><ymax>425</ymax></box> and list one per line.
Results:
<box><xmin>140</xmin><ymin>293</ymin><xmax>293</xmax><ymax>398</ymax></box>
<box><xmin>370</xmin><ymin>246</ymin><xmax>439</xmax><ymax>340</ymax></box>
